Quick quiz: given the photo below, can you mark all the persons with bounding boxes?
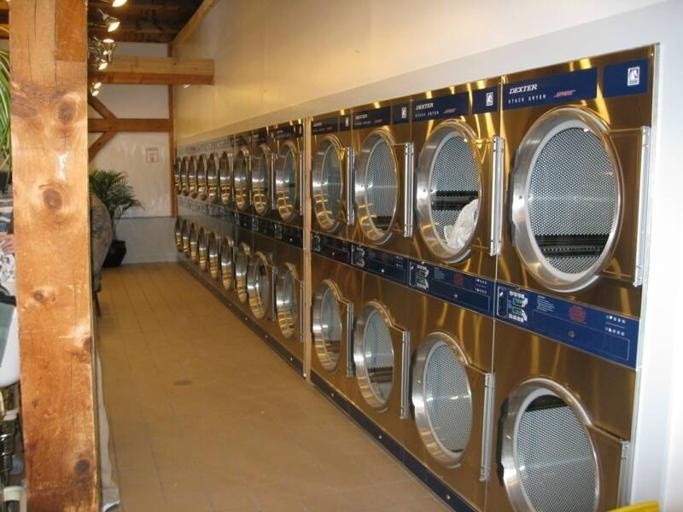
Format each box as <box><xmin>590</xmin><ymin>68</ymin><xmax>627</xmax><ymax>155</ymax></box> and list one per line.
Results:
<box><xmin>0</xmin><ymin>184</ymin><xmax>121</xmax><ymax>512</ymax></box>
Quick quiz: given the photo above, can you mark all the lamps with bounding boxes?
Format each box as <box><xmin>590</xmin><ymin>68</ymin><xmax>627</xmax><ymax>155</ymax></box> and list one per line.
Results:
<box><xmin>87</xmin><ymin>1</ymin><xmax>127</xmax><ymax>98</ymax></box>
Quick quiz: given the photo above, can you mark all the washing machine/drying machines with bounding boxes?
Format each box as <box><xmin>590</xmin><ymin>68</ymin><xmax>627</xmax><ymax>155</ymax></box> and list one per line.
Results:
<box><xmin>175</xmin><ymin>118</ymin><xmax>306</xmax><ymax>377</ymax></box>
<box><xmin>309</xmin><ymin>44</ymin><xmax>662</xmax><ymax>510</ymax></box>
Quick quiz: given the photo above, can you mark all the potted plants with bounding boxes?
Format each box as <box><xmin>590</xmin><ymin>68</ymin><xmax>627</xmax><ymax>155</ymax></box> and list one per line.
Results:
<box><xmin>88</xmin><ymin>169</ymin><xmax>149</xmax><ymax>268</ymax></box>
